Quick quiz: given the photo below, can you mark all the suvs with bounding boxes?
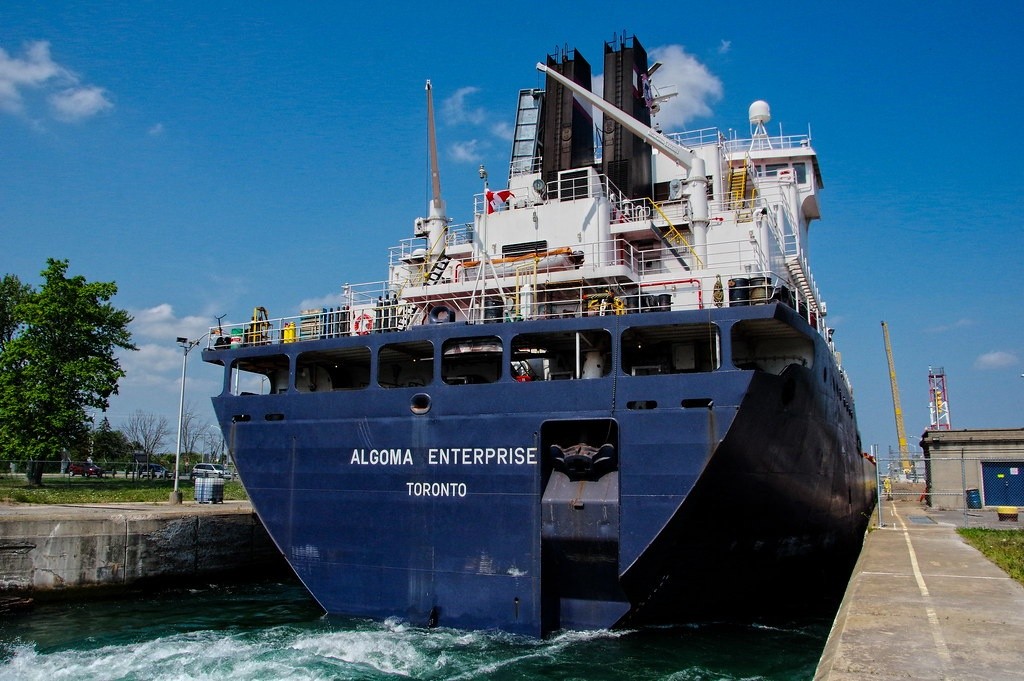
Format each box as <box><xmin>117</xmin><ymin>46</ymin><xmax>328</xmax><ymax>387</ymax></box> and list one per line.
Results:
<box><xmin>192</xmin><ymin>463</ymin><xmax>233</xmax><ymax>480</ymax></box>
<box><xmin>68</xmin><ymin>462</ymin><xmax>101</xmax><ymax>477</ymax></box>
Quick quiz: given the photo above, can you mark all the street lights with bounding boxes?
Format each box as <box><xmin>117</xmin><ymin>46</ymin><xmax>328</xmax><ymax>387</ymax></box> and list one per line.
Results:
<box><xmin>909</xmin><ymin>435</ymin><xmax>924</xmax><ymax>455</ymax></box>
<box><xmin>173</xmin><ymin>330</ymin><xmax>217</xmax><ymax>491</ymax></box>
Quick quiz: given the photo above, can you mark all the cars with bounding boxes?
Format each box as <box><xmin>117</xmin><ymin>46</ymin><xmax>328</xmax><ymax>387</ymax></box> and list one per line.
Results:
<box><xmin>140</xmin><ymin>464</ymin><xmax>172</xmax><ymax>478</ymax></box>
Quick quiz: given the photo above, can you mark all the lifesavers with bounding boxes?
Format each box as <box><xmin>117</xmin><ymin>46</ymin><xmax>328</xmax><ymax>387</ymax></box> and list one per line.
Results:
<box><xmin>354</xmin><ymin>313</ymin><xmax>373</xmax><ymax>336</ymax></box>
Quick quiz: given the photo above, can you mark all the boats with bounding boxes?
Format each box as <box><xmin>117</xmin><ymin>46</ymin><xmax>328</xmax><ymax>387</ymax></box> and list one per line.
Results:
<box><xmin>202</xmin><ymin>28</ymin><xmax>876</xmax><ymax>640</ymax></box>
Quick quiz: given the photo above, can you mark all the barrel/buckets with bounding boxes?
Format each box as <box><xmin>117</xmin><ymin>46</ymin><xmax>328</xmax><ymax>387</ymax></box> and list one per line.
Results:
<box><xmin>750</xmin><ymin>277</ymin><xmax>772</xmax><ymax>305</ymax></box>
<box><xmin>485</xmin><ymin>301</ymin><xmax>504</xmax><ymax>323</ymax></box>
<box><xmin>966</xmin><ymin>489</ymin><xmax>982</xmax><ymax>509</ymax></box>
<box><xmin>627</xmin><ymin>297</ymin><xmax>638</xmax><ymax>314</ymax></box>
<box><xmin>230</xmin><ymin>329</ymin><xmax>246</xmax><ymax>349</ymax></box>
<box><xmin>658</xmin><ymin>294</ymin><xmax>671</xmax><ymax>312</ymax></box>
<box><xmin>728</xmin><ymin>278</ymin><xmax>750</xmax><ymax>307</ymax></box>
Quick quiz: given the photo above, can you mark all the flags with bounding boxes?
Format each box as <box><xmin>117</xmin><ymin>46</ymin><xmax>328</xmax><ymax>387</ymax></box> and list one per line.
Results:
<box><xmin>487</xmin><ymin>189</ymin><xmax>515</xmax><ymax>213</ymax></box>
